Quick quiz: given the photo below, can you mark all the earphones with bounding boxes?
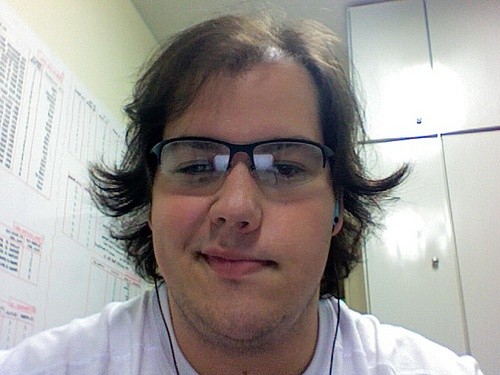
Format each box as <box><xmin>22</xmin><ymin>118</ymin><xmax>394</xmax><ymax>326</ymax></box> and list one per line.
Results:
<box><xmin>333</xmin><ymin>200</ymin><xmax>339</xmax><ymax>225</ymax></box>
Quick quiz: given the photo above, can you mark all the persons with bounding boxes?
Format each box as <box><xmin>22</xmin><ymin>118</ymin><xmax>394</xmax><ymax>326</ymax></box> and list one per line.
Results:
<box><xmin>0</xmin><ymin>14</ymin><xmax>486</xmax><ymax>375</ymax></box>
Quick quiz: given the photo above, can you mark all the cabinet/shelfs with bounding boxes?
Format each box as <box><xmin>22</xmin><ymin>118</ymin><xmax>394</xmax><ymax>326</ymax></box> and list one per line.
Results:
<box><xmin>354</xmin><ymin>127</ymin><xmax>499</xmax><ymax>375</ymax></box>
<box><xmin>346</xmin><ymin>0</ymin><xmax>500</xmax><ymax>143</ymax></box>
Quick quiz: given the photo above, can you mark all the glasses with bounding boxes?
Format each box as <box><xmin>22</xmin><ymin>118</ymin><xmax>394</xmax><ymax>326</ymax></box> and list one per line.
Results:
<box><xmin>151</xmin><ymin>136</ymin><xmax>337</xmax><ymax>188</ymax></box>
